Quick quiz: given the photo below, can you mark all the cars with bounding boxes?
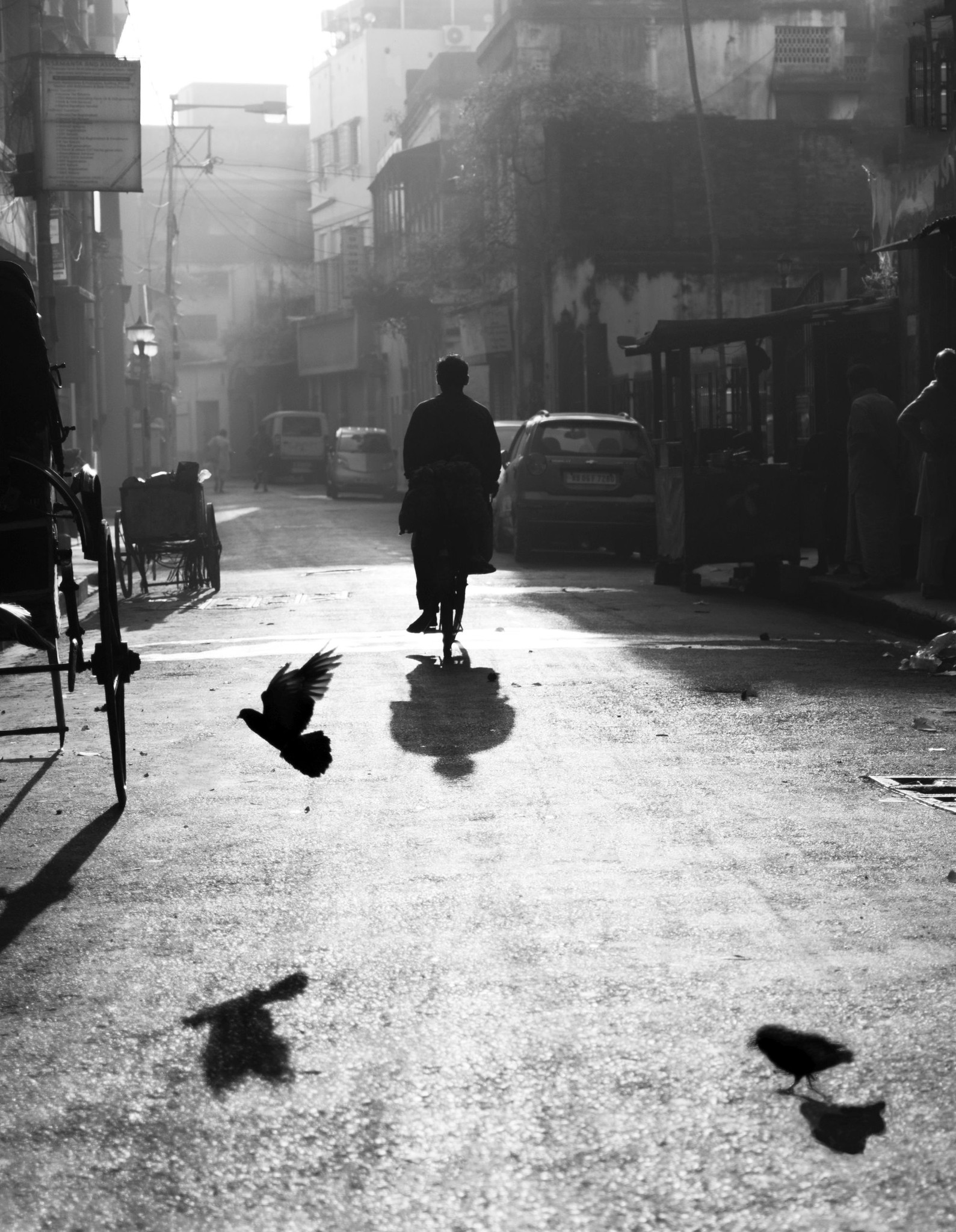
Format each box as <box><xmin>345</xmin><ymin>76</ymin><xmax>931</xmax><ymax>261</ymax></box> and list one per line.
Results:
<box><xmin>489</xmin><ymin>419</ymin><xmax>595</xmax><ymax>486</ymax></box>
<box><xmin>329</xmin><ymin>427</ymin><xmax>400</xmax><ymax>499</ymax></box>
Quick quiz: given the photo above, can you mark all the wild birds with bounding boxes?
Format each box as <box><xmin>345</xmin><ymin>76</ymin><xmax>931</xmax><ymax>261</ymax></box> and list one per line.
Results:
<box><xmin>236</xmin><ymin>641</ymin><xmax>342</xmax><ymax>779</ymax></box>
<box><xmin>749</xmin><ymin>1025</ymin><xmax>855</xmax><ymax>1100</ymax></box>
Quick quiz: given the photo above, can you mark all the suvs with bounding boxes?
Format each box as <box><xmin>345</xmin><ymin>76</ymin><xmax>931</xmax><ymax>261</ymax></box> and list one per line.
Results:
<box><xmin>492</xmin><ymin>408</ymin><xmax>659</xmax><ymax>564</ymax></box>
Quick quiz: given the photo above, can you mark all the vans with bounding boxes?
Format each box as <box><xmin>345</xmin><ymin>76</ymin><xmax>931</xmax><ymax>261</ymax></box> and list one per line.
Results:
<box><xmin>260</xmin><ymin>410</ymin><xmax>330</xmax><ymax>475</ymax></box>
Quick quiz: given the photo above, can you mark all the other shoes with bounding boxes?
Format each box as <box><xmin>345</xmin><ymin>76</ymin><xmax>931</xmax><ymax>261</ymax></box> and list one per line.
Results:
<box><xmin>407</xmin><ymin>610</ymin><xmax>437</xmax><ymax>632</ymax></box>
<box><xmin>468</xmin><ymin>563</ymin><xmax>496</xmax><ymax>574</ymax></box>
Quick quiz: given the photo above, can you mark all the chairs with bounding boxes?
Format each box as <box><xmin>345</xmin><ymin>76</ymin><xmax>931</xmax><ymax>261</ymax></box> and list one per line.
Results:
<box><xmin>597</xmin><ymin>437</ymin><xmax>620</xmax><ymax>453</ymax></box>
<box><xmin>541</xmin><ymin>436</ymin><xmax>561</xmax><ymax>453</ymax></box>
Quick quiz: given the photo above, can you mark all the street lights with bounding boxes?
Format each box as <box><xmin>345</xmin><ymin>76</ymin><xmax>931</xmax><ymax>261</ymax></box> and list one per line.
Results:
<box><xmin>163</xmin><ymin>100</ymin><xmax>288</xmax><ymax>296</ymax></box>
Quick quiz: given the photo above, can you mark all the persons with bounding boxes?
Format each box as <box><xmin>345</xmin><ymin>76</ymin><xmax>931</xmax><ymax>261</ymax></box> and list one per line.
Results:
<box><xmin>245</xmin><ymin>423</ymin><xmax>277</xmax><ymax>492</ymax></box>
<box><xmin>895</xmin><ymin>346</ymin><xmax>956</xmax><ymax>598</ymax></box>
<box><xmin>848</xmin><ymin>374</ymin><xmax>896</xmax><ymax>590</ymax></box>
<box><xmin>207</xmin><ymin>428</ymin><xmax>231</xmax><ymax>492</ymax></box>
<box><xmin>397</xmin><ymin>356</ymin><xmax>503</xmax><ymax>636</ymax></box>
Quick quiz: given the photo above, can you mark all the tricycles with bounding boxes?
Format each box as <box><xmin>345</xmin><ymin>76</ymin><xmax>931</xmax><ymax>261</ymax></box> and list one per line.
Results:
<box><xmin>1</xmin><ymin>361</ymin><xmax>142</xmax><ymax>809</ymax></box>
<box><xmin>114</xmin><ymin>461</ymin><xmax>224</xmax><ymax>595</ymax></box>
